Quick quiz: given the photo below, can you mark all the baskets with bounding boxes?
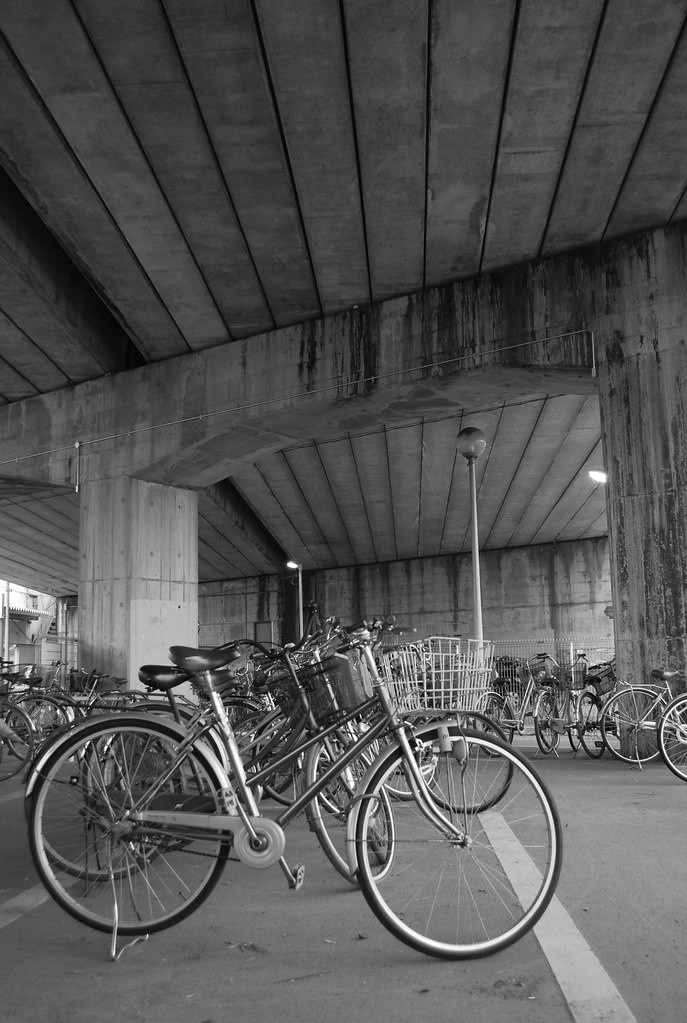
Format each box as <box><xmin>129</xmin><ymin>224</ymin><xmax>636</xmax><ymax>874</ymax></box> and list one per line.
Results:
<box><xmin>265</xmin><ymin>631</ymin><xmax>620</xmax><ymax>730</ymax></box>
<box><xmin>31</xmin><ymin>664</ymin><xmax>58</xmax><ymax>688</ymax></box>
<box><xmin>69</xmin><ymin>668</ymin><xmax>99</xmax><ymax>692</ymax></box>
<box><xmin>0</xmin><ymin>674</ymin><xmax>9</xmax><ymax>696</ymax></box>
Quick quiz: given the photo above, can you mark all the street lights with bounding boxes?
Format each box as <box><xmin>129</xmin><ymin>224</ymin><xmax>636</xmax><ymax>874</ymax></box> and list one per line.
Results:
<box><xmin>455</xmin><ymin>423</ymin><xmax>490</xmax><ymax>697</ymax></box>
<box><xmin>283</xmin><ymin>560</ymin><xmax>305</xmax><ymax>646</ymax></box>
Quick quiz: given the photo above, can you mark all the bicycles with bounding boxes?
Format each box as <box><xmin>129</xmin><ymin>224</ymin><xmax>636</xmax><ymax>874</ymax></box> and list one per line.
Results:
<box><xmin>0</xmin><ymin>599</ymin><xmax>686</xmax><ymax>963</ymax></box>
